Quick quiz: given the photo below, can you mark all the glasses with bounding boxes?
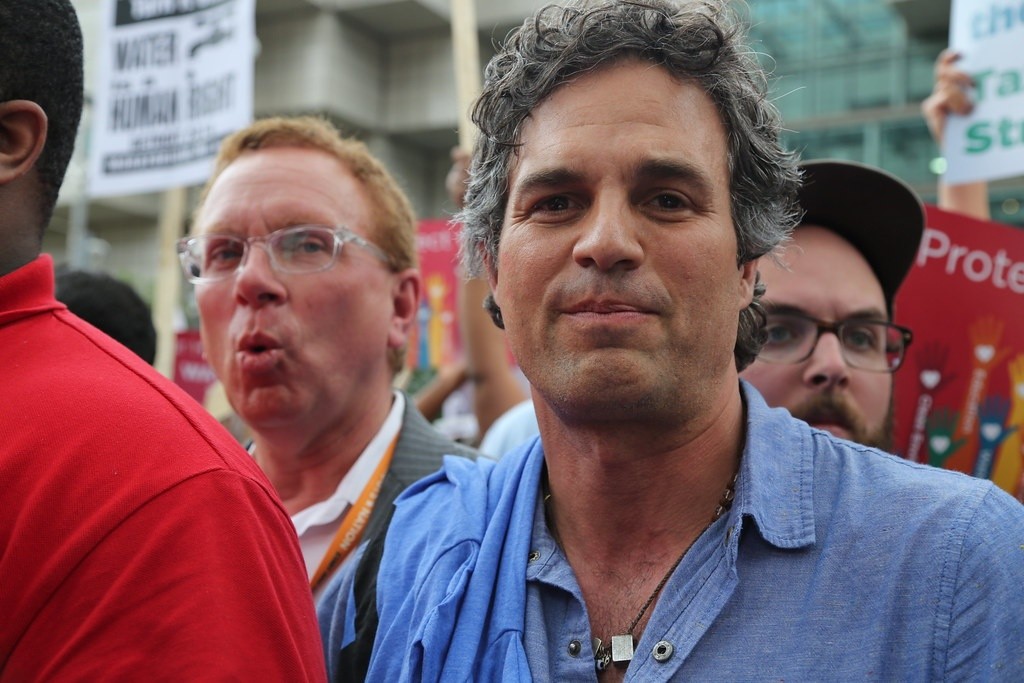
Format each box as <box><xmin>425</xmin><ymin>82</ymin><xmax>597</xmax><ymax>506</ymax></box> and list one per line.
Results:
<box><xmin>755</xmin><ymin>311</ymin><xmax>913</xmax><ymax>373</ymax></box>
<box><xmin>174</xmin><ymin>225</ymin><xmax>396</xmax><ymax>285</ymax></box>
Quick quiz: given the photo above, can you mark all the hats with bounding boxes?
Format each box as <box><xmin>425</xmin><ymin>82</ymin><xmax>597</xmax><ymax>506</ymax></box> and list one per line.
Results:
<box><xmin>784</xmin><ymin>160</ymin><xmax>925</xmax><ymax>317</ymax></box>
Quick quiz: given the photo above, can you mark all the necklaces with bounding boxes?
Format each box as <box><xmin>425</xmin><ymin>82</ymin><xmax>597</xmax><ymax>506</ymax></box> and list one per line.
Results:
<box><xmin>541</xmin><ymin>447</ymin><xmax>744</xmax><ymax>670</ymax></box>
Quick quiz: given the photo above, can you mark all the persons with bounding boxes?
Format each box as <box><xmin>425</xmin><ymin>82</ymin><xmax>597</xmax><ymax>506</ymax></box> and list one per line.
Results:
<box><xmin>0</xmin><ymin>2</ymin><xmax>331</xmax><ymax>683</ymax></box>
<box><xmin>53</xmin><ymin>269</ymin><xmax>157</xmax><ymax>370</ymax></box>
<box><xmin>922</xmin><ymin>46</ymin><xmax>989</xmax><ymax>221</ymax></box>
<box><xmin>189</xmin><ymin>116</ymin><xmax>496</xmax><ymax>617</ymax></box>
<box><xmin>410</xmin><ymin>147</ymin><xmax>534</xmax><ymax>447</ymax></box>
<box><xmin>316</xmin><ymin>1</ymin><xmax>1024</xmax><ymax>683</ymax></box>
<box><xmin>739</xmin><ymin>162</ymin><xmax>923</xmax><ymax>441</ymax></box>
